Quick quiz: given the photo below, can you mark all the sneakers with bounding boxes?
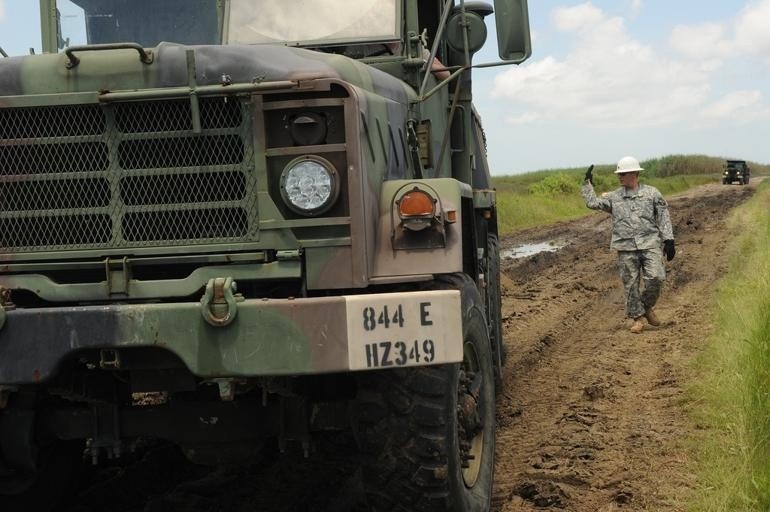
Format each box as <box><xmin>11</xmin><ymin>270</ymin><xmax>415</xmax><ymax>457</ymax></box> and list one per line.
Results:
<box><xmin>645</xmin><ymin>307</ymin><xmax>659</xmax><ymax>326</ymax></box>
<box><xmin>631</xmin><ymin>317</ymin><xmax>644</xmax><ymax>334</ymax></box>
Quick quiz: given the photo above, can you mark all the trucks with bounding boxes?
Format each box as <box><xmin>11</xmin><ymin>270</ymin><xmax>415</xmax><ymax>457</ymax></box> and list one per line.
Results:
<box><xmin>0</xmin><ymin>1</ymin><xmax>535</xmax><ymax>511</ymax></box>
<box><xmin>721</xmin><ymin>160</ymin><xmax>751</xmax><ymax>185</ymax></box>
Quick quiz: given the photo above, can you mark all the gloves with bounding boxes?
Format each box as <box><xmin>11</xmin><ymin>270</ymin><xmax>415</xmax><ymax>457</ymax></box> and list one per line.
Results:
<box><xmin>663</xmin><ymin>239</ymin><xmax>675</xmax><ymax>261</ymax></box>
<box><xmin>585</xmin><ymin>165</ymin><xmax>595</xmax><ymax>186</ymax></box>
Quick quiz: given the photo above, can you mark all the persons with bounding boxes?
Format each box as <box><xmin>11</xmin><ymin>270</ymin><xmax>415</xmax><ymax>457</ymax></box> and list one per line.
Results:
<box><xmin>582</xmin><ymin>156</ymin><xmax>677</xmax><ymax>333</ymax></box>
<box><xmin>343</xmin><ymin>19</ymin><xmax>451</xmax><ymax>80</ymax></box>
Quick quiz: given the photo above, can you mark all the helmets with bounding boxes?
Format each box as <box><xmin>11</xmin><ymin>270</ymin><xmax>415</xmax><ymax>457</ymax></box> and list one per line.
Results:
<box><xmin>614</xmin><ymin>156</ymin><xmax>645</xmax><ymax>173</ymax></box>
